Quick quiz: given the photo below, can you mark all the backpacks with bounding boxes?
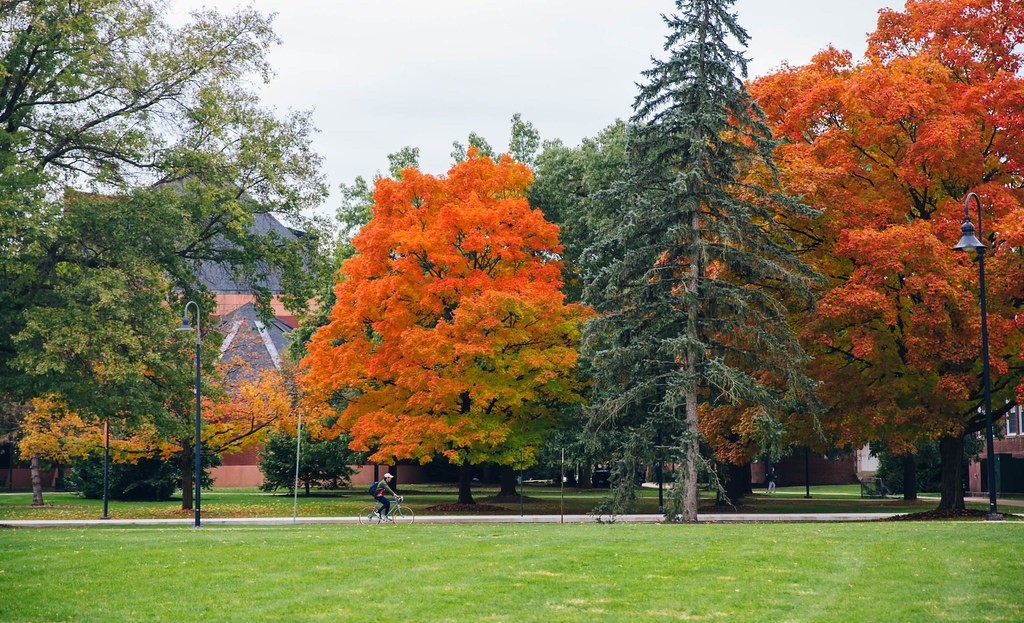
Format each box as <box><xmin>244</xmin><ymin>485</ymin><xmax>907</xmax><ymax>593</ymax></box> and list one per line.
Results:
<box><xmin>369</xmin><ymin>480</ymin><xmax>387</xmax><ymax>496</ymax></box>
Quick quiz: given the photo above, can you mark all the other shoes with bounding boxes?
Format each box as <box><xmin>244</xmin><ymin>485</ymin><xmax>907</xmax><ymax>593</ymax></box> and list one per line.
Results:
<box><xmin>383</xmin><ymin>517</ymin><xmax>392</xmax><ymax>522</ymax></box>
<box><xmin>374</xmin><ymin>511</ymin><xmax>382</xmax><ymax>519</ymax></box>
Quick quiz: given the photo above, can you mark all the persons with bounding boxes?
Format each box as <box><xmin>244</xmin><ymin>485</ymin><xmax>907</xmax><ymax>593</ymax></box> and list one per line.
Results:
<box><xmin>768</xmin><ymin>467</ymin><xmax>777</xmax><ymax>493</ymax></box>
<box><xmin>374</xmin><ymin>473</ymin><xmax>400</xmax><ymax>522</ymax></box>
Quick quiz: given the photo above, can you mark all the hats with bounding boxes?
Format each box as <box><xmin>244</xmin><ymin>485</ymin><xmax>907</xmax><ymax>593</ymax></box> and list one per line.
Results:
<box><xmin>384</xmin><ymin>473</ymin><xmax>394</xmax><ymax>478</ymax></box>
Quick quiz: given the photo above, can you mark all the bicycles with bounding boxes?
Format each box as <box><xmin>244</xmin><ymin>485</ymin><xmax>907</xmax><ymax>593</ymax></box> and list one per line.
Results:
<box><xmin>358</xmin><ymin>496</ymin><xmax>415</xmax><ymax>525</ymax></box>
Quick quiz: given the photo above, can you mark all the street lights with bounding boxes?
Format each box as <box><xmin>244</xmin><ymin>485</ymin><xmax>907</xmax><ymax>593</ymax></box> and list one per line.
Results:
<box><xmin>173</xmin><ymin>301</ymin><xmax>202</xmax><ymax>526</ymax></box>
<box><xmin>950</xmin><ymin>190</ymin><xmax>1003</xmax><ymax>520</ymax></box>
